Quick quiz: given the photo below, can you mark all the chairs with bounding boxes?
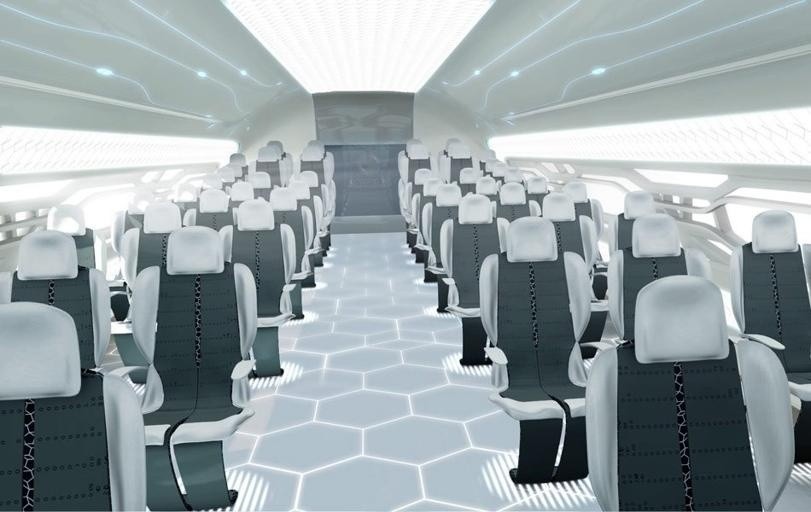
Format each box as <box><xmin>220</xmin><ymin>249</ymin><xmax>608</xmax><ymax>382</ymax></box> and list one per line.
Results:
<box><xmin>0</xmin><ymin>140</ymin><xmax>336</xmax><ymax>511</ymax></box>
<box><xmin>398</xmin><ymin>138</ymin><xmax>811</xmax><ymax>511</ymax></box>
